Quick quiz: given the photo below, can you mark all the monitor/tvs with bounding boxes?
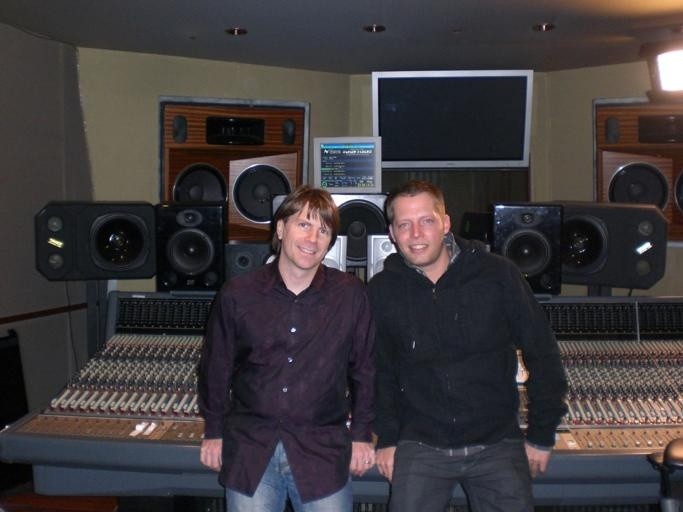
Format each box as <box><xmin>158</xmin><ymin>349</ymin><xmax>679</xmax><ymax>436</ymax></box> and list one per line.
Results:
<box><xmin>316</xmin><ymin>136</ymin><xmax>381</xmax><ymax>195</ymax></box>
<box><xmin>372</xmin><ymin>71</ymin><xmax>533</xmax><ymax>170</ymax></box>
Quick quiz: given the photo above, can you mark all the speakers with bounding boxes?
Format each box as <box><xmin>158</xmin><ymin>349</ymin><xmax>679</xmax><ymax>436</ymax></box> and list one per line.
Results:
<box><xmin>223</xmin><ymin>244</ymin><xmax>273</xmax><ymax>282</ymax></box>
<box><xmin>493</xmin><ymin>202</ymin><xmax>563</xmax><ymax>299</ymax></box>
<box><xmin>158</xmin><ymin>199</ymin><xmax>221</xmax><ymax>291</ymax></box>
<box><xmin>554</xmin><ymin>200</ymin><xmax>669</xmax><ymax>291</ymax></box>
<box><xmin>267</xmin><ymin>193</ymin><xmax>393</xmax><ymax>266</ymax></box>
<box><xmin>318</xmin><ymin>236</ymin><xmax>345</xmax><ymax>272</ymax></box>
<box><xmin>157</xmin><ymin>98</ymin><xmax>308</xmax><ymax>205</ymax></box>
<box><xmin>597</xmin><ymin>105</ymin><xmax>683</xmax><ymax>246</ymax></box>
<box><xmin>367</xmin><ymin>234</ymin><xmax>396</xmax><ymax>283</ymax></box>
<box><xmin>230</xmin><ymin>154</ymin><xmax>297</xmax><ymax>240</ymax></box>
<box><xmin>35</xmin><ymin>203</ymin><xmax>157</xmax><ymax>280</ymax></box>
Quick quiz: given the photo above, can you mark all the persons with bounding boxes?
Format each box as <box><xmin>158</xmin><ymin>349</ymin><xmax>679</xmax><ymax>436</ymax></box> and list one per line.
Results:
<box><xmin>363</xmin><ymin>179</ymin><xmax>569</xmax><ymax>512</ymax></box>
<box><xmin>196</xmin><ymin>183</ymin><xmax>379</xmax><ymax>511</ymax></box>
